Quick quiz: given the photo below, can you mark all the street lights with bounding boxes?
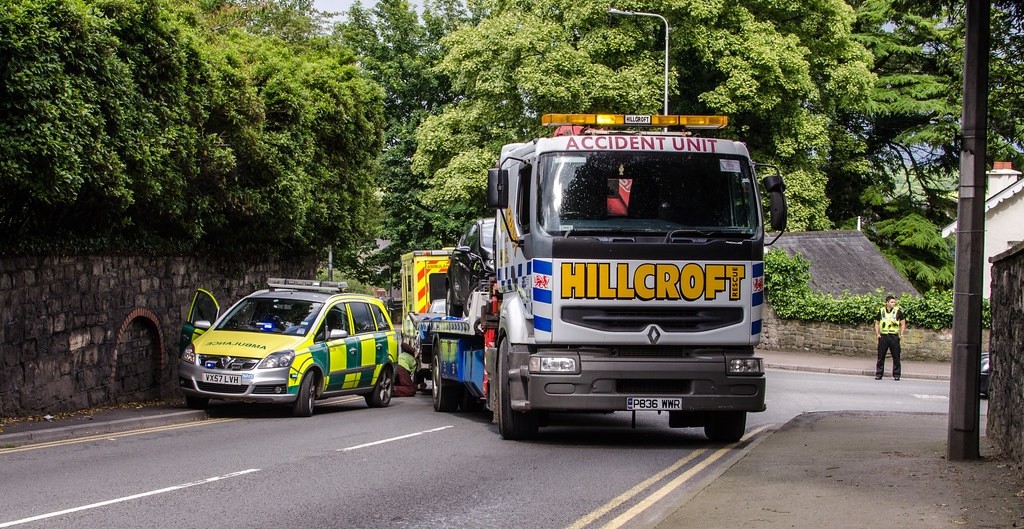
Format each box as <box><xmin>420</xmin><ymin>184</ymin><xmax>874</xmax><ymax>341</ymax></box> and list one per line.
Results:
<box><xmin>605</xmin><ymin>7</ymin><xmax>669</xmax><ymax>132</ymax></box>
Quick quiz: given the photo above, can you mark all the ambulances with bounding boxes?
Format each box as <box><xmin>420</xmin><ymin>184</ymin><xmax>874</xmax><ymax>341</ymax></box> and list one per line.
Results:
<box><xmin>178</xmin><ymin>278</ymin><xmax>398</xmax><ymax>417</ymax></box>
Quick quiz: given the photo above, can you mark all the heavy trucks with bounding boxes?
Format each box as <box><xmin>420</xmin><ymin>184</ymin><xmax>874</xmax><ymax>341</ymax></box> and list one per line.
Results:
<box><xmin>409</xmin><ymin>114</ymin><xmax>787</xmax><ymax>446</ymax></box>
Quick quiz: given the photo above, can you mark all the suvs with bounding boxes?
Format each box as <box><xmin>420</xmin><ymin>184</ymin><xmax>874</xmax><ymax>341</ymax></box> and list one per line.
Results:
<box><xmin>444</xmin><ymin>217</ymin><xmax>498</xmax><ymax>318</ymax></box>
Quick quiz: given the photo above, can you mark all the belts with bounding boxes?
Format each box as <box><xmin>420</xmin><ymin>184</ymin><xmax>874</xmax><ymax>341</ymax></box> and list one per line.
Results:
<box><xmin>883</xmin><ymin>334</ymin><xmax>896</xmax><ymax>337</ymax></box>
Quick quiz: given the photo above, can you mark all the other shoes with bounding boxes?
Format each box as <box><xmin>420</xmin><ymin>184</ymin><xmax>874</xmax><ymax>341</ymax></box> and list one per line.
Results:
<box><xmin>894</xmin><ymin>378</ymin><xmax>899</xmax><ymax>380</ymax></box>
<box><xmin>875</xmin><ymin>377</ymin><xmax>882</xmax><ymax>380</ymax></box>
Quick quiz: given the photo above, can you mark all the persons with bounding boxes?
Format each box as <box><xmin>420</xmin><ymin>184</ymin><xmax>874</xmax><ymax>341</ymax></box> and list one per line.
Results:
<box><xmin>391</xmin><ymin>343</ymin><xmax>420</xmax><ymax>397</ymax></box>
<box><xmin>874</xmin><ymin>296</ymin><xmax>906</xmax><ymax>381</ymax></box>
